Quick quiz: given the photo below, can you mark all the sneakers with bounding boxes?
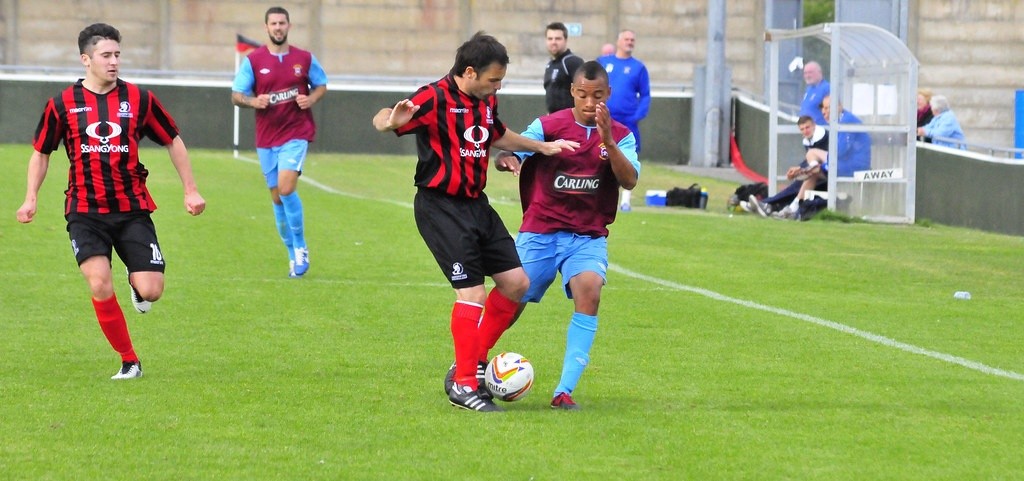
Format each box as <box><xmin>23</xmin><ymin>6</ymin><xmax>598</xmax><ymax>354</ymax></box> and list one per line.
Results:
<box><xmin>550</xmin><ymin>392</ymin><xmax>578</xmax><ymax>409</ymax></box>
<box><xmin>444</xmin><ymin>361</ymin><xmax>456</xmax><ymax>395</ymax></box>
<box><xmin>770</xmin><ymin>205</ymin><xmax>796</xmax><ymax>221</ymax></box>
<box><xmin>740</xmin><ymin>200</ymin><xmax>755</xmax><ymax>213</ymax></box>
<box><xmin>621</xmin><ymin>203</ymin><xmax>630</xmax><ymax>211</ymax></box>
<box><xmin>111</xmin><ymin>360</ymin><xmax>143</xmax><ymax>380</ymax></box>
<box><xmin>294</xmin><ymin>246</ymin><xmax>310</xmax><ymax>275</ymax></box>
<box><xmin>448</xmin><ymin>381</ymin><xmax>507</xmax><ymax>412</ymax></box>
<box><xmin>289</xmin><ymin>260</ymin><xmax>302</xmax><ymax>278</ymax></box>
<box><xmin>125</xmin><ymin>265</ymin><xmax>152</xmax><ymax>313</ymax></box>
<box><xmin>475</xmin><ymin>361</ymin><xmax>494</xmax><ymax>399</ymax></box>
<box><xmin>749</xmin><ymin>194</ymin><xmax>773</xmax><ymax>219</ymax></box>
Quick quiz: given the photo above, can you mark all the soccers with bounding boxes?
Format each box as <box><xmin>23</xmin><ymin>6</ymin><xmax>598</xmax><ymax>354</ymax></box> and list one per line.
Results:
<box><xmin>485</xmin><ymin>352</ymin><xmax>534</xmax><ymax>401</ymax></box>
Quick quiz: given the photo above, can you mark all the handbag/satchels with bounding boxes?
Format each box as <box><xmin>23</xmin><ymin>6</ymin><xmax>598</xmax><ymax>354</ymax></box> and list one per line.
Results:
<box><xmin>794</xmin><ymin>195</ymin><xmax>829</xmax><ymax>221</ymax></box>
<box><xmin>685</xmin><ymin>183</ymin><xmax>701</xmax><ymax>207</ymax></box>
<box><xmin>645</xmin><ymin>190</ymin><xmax>666</xmax><ymax>205</ymax></box>
<box><xmin>666</xmin><ymin>188</ymin><xmax>687</xmax><ymax>206</ymax></box>
<box><xmin>734</xmin><ymin>183</ymin><xmax>768</xmax><ymax>200</ymax></box>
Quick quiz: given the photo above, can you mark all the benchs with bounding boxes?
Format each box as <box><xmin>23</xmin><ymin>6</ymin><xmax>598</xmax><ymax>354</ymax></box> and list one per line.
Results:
<box><xmin>837</xmin><ymin>177</ymin><xmax>907</xmax><ymax>216</ymax></box>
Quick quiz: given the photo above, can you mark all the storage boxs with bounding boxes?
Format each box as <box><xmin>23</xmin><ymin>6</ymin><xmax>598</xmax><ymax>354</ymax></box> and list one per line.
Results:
<box><xmin>646</xmin><ymin>190</ymin><xmax>667</xmax><ymax>206</ymax></box>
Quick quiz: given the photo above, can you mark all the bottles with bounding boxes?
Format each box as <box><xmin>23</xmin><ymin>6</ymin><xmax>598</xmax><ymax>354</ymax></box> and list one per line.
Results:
<box><xmin>700</xmin><ymin>192</ymin><xmax>708</xmax><ymax>209</ymax></box>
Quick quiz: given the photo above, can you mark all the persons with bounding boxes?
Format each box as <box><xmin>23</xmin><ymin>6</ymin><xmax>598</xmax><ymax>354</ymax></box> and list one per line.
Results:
<box><xmin>918</xmin><ymin>96</ymin><xmax>966</xmax><ymax>149</ymax></box>
<box><xmin>740</xmin><ymin>116</ymin><xmax>829</xmax><ymax>217</ymax></box>
<box><xmin>799</xmin><ymin>61</ymin><xmax>831</xmax><ymax>126</ymax></box>
<box><xmin>596</xmin><ymin>30</ymin><xmax>651</xmax><ymax>213</ymax></box>
<box><xmin>771</xmin><ymin>94</ymin><xmax>872</xmax><ymax>220</ymax></box>
<box><xmin>543</xmin><ymin>23</ymin><xmax>584</xmax><ymax>114</ymax></box>
<box><xmin>231</xmin><ymin>7</ymin><xmax>328</xmax><ymax>277</ymax></box>
<box><xmin>16</xmin><ymin>23</ymin><xmax>206</xmax><ymax>379</ymax></box>
<box><xmin>917</xmin><ymin>89</ymin><xmax>934</xmax><ymax>144</ymax></box>
<box><xmin>444</xmin><ymin>60</ymin><xmax>641</xmax><ymax>410</ymax></box>
<box><xmin>373</xmin><ymin>30</ymin><xmax>580</xmax><ymax>412</ymax></box>
